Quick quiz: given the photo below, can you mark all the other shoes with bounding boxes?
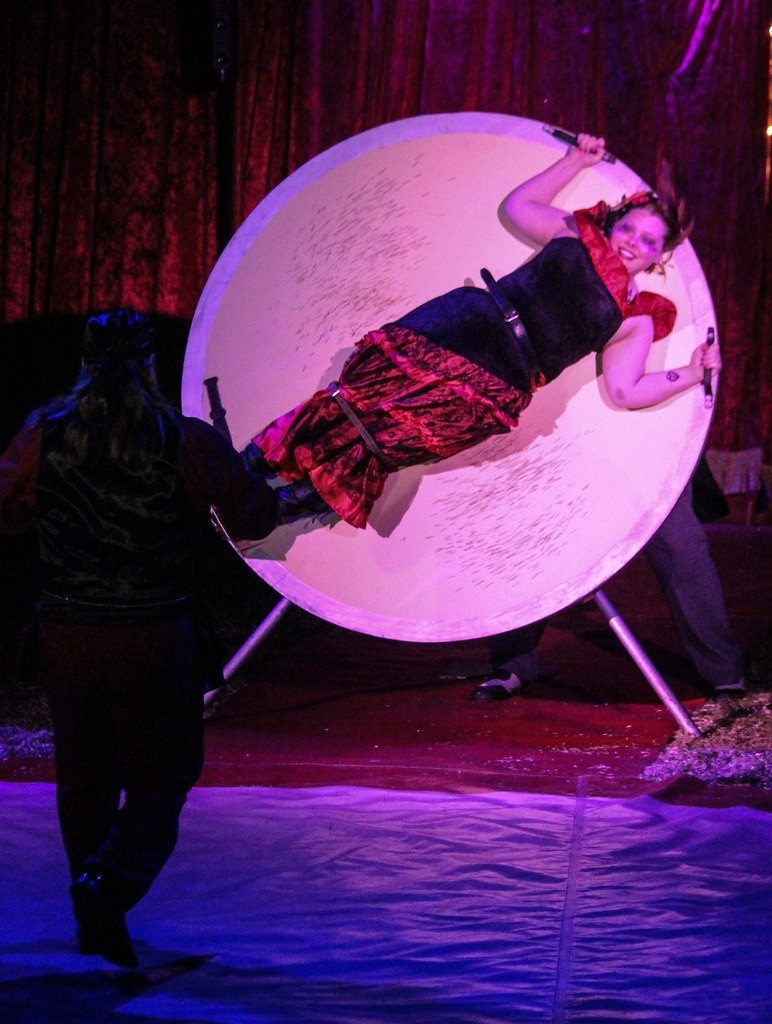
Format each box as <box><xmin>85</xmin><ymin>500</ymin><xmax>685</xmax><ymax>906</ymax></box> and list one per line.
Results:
<box><xmin>716</xmin><ymin>677</ymin><xmax>747</xmax><ymax>703</ymax></box>
<box><xmin>69</xmin><ymin>869</ymin><xmax>139</xmax><ymax>970</ymax></box>
<box><xmin>474</xmin><ymin>673</ymin><xmax>533</xmax><ymax>702</ymax></box>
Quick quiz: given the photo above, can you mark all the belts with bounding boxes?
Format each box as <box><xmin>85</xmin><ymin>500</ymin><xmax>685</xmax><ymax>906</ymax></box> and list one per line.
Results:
<box><xmin>480</xmin><ymin>268</ymin><xmax>542</xmax><ymax>385</ymax></box>
<box><xmin>329</xmin><ymin>381</ymin><xmax>398</xmax><ymax>475</ymax></box>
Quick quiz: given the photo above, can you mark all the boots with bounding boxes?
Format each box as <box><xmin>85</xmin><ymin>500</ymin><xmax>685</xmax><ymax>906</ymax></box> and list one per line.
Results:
<box><xmin>274</xmin><ymin>474</ymin><xmax>325</xmax><ymax>525</ymax></box>
<box><xmin>239</xmin><ymin>443</ymin><xmax>278</xmax><ymax>480</ymax></box>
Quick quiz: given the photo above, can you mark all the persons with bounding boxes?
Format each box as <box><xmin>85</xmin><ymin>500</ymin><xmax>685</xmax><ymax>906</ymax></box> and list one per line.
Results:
<box><xmin>215</xmin><ymin>135</ymin><xmax>720</xmax><ymax>543</ymax></box>
<box><xmin>0</xmin><ymin>309</ymin><xmax>279</xmax><ymax>969</ymax></box>
<box><xmin>470</xmin><ymin>451</ymin><xmax>744</xmax><ymax>702</ymax></box>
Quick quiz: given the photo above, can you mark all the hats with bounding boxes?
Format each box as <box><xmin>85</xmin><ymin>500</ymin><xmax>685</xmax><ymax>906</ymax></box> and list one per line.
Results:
<box><xmin>84</xmin><ymin>308</ymin><xmax>157</xmax><ymax>364</ymax></box>
